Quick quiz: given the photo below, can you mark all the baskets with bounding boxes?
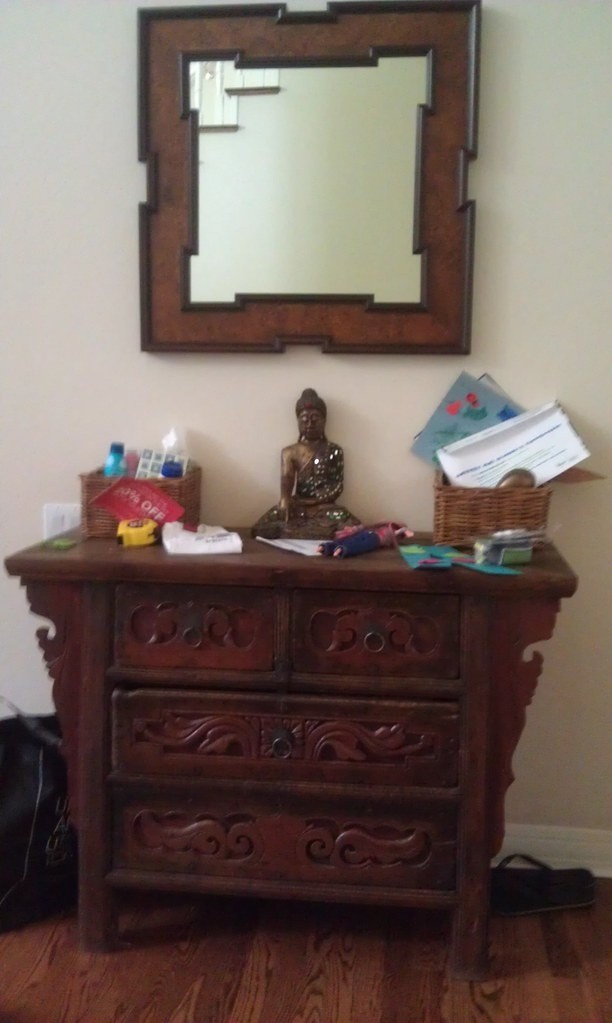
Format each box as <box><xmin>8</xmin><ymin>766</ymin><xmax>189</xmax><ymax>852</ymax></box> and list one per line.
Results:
<box><xmin>433</xmin><ymin>469</ymin><xmax>553</xmax><ymax>551</ymax></box>
<box><xmin>77</xmin><ymin>461</ymin><xmax>202</xmax><ymax>539</ymax></box>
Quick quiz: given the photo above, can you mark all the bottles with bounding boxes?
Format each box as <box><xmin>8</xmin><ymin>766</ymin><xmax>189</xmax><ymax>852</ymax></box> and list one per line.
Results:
<box><xmin>157</xmin><ymin>460</ymin><xmax>182</xmax><ymax>481</ymax></box>
<box><xmin>104</xmin><ymin>442</ymin><xmax>127</xmax><ymax>478</ymax></box>
<box><xmin>122</xmin><ymin>448</ymin><xmax>139</xmax><ymax>479</ymax></box>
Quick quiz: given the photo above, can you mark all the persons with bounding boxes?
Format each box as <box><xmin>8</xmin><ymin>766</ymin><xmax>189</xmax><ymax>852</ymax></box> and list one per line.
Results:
<box><xmin>250</xmin><ymin>388</ymin><xmax>364</xmax><ymax>541</ymax></box>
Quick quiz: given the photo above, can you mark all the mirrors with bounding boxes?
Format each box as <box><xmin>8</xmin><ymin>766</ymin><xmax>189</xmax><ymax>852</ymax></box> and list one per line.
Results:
<box><xmin>132</xmin><ymin>0</ymin><xmax>484</xmax><ymax>358</ymax></box>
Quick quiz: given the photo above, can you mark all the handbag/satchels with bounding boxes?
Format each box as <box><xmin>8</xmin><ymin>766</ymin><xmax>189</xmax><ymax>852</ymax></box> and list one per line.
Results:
<box><xmin>491</xmin><ymin>852</ymin><xmax>595</xmax><ymax>917</ymax></box>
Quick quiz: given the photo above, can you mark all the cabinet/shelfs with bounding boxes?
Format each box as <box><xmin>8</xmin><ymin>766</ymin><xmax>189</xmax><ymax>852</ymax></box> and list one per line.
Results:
<box><xmin>3</xmin><ymin>528</ymin><xmax>585</xmax><ymax>982</ymax></box>
